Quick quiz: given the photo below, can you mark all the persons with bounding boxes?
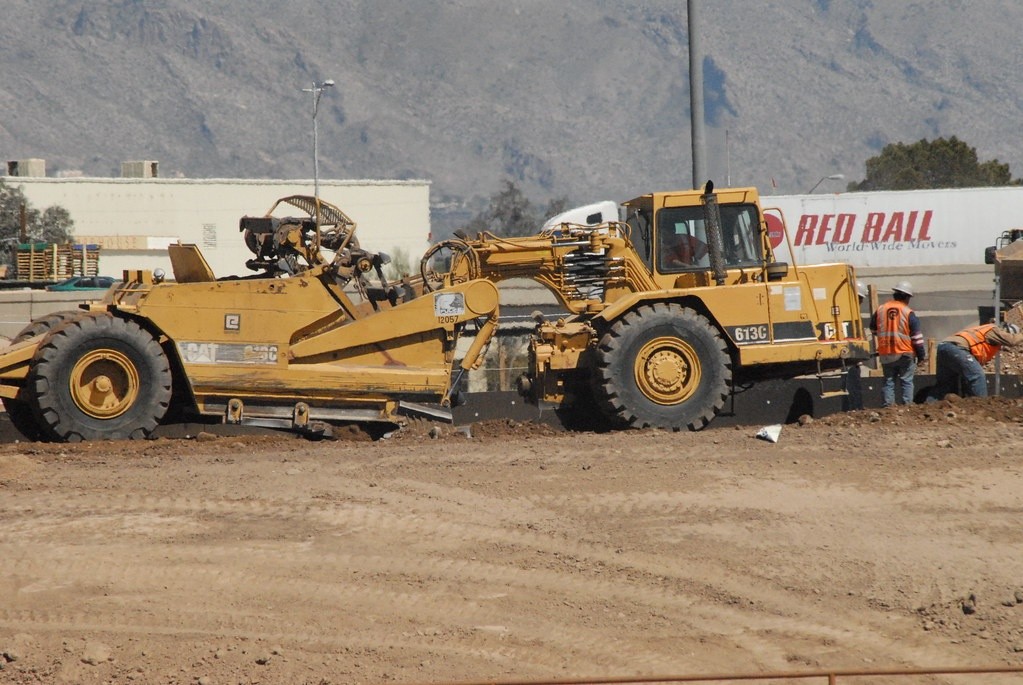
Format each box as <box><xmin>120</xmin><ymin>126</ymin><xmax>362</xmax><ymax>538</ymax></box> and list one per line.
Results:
<box><xmin>870</xmin><ymin>281</ymin><xmax>926</xmax><ymax>408</ymax></box>
<box><xmin>924</xmin><ymin>324</ymin><xmax>1023</xmax><ymax>405</ymax></box>
<box><xmin>661</xmin><ymin>218</ymin><xmax>711</xmax><ymax>269</ymax></box>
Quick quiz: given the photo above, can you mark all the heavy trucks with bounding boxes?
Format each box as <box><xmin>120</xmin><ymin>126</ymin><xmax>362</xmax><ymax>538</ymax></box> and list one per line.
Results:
<box><xmin>539</xmin><ymin>186</ymin><xmax>1023</xmax><ymax>271</ymax></box>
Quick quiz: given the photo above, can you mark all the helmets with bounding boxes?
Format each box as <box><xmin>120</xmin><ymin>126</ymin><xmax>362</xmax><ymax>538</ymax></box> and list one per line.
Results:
<box><xmin>891</xmin><ymin>281</ymin><xmax>913</xmax><ymax>296</ymax></box>
<box><xmin>1005</xmin><ymin>323</ymin><xmax>1019</xmax><ymax>335</ymax></box>
<box><xmin>857</xmin><ymin>281</ymin><xmax>866</xmax><ymax>299</ymax></box>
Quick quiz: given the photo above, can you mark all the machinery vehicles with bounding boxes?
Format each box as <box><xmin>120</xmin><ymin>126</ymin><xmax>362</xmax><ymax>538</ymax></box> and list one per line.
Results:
<box><xmin>0</xmin><ymin>182</ymin><xmax>882</xmax><ymax>441</ymax></box>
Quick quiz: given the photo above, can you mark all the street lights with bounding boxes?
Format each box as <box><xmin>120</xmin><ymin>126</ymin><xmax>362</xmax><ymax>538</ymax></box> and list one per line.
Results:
<box><xmin>302</xmin><ymin>80</ymin><xmax>333</xmax><ymax>198</ymax></box>
